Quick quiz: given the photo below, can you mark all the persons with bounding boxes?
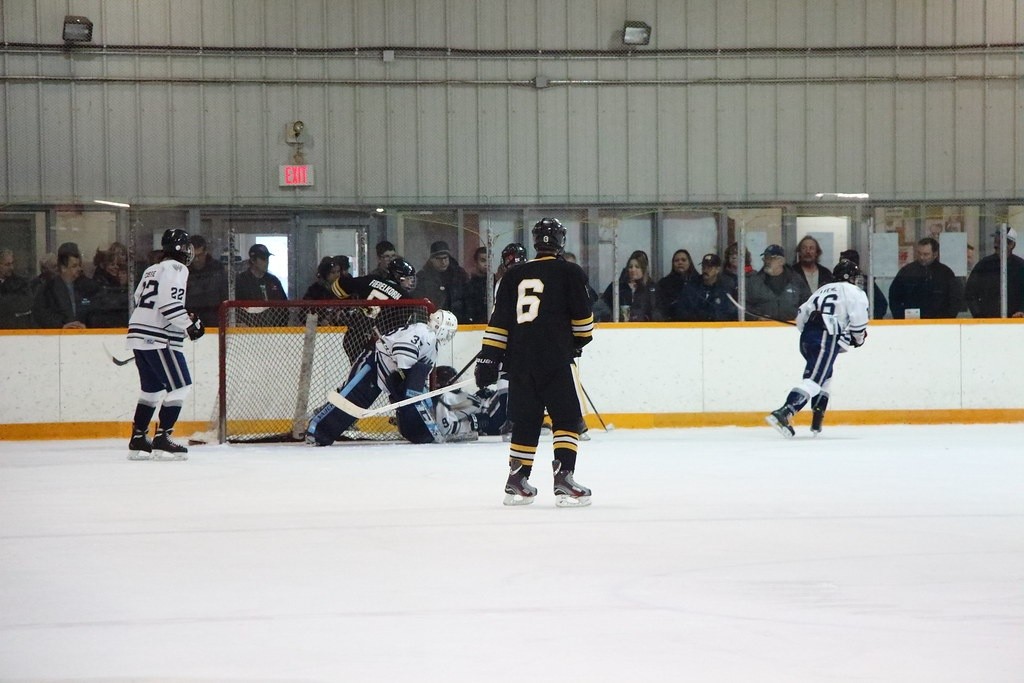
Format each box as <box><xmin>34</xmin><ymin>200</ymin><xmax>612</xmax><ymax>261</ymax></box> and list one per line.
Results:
<box><xmin>957</xmin><ymin>245</ymin><xmax>975</xmax><ymax>311</ymax></box>
<box><xmin>766</xmin><ymin>257</ymin><xmax>869</xmax><ymax>438</ymax></box>
<box><xmin>0</xmin><ymin>234</ymin><xmax>841</xmax><ymax>328</ymax></box>
<box><xmin>840</xmin><ymin>250</ymin><xmax>888</xmax><ymax>319</ymax></box>
<box><xmin>430</xmin><ymin>366</ymin><xmax>552</xmax><ymax>443</ymax></box>
<box><xmin>302</xmin><ymin>309</ymin><xmax>457</xmax><ymax>444</ymax></box>
<box><xmin>126</xmin><ymin>228</ymin><xmax>204</xmax><ymax>459</ymax></box>
<box><xmin>888</xmin><ymin>238</ymin><xmax>959</xmax><ymax>319</ymax></box>
<box><xmin>963</xmin><ymin>227</ymin><xmax>1024</xmax><ymax>319</ymax></box>
<box><xmin>313</xmin><ymin>257</ymin><xmax>416</xmax><ymax>430</ymax></box>
<box><xmin>474</xmin><ymin>218</ymin><xmax>593</xmax><ymax>506</ymax></box>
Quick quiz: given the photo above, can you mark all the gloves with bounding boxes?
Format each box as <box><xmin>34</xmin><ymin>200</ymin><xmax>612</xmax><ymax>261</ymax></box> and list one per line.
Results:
<box><xmin>187</xmin><ymin>315</ymin><xmax>204</xmax><ymax>340</ymax></box>
<box><xmin>850</xmin><ymin>330</ymin><xmax>867</xmax><ymax>348</ymax></box>
<box><xmin>475</xmin><ymin>357</ymin><xmax>499</xmax><ymax>389</ymax></box>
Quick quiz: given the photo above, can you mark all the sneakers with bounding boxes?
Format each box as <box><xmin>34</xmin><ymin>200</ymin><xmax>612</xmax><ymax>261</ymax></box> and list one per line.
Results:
<box><xmin>552</xmin><ymin>460</ymin><xmax>592</xmax><ymax>507</ymax></box>
<box><xmin>127</xmin><ymin>428</ymin><xmax>152</xmax><ymax>460</ymax></box>
<box><xmin>503</xmin><ymin>458</ymin><xmax>537</xmax><ymax>505</ymax></box>
<box><xmin>152</xmin><ymin>429</ymin><xmax>188</xmax><ymax>461</ymax></box>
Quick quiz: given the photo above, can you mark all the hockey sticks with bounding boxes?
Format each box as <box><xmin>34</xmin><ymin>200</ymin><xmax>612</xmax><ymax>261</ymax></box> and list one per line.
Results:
<box><xmin>443</xmin><ymin>350</ymin><xmax>482</xmax><ymax>386</ymax></box>
<box><xmin>725</xmin><ymin>292</ymin><xmax>853</xmax><ymax>345</ymax></box>
<box><xmin>326</xmin><ymin>370</ymin><xmax>507</xmax><ymax>419</ymax></box>
<box><xmin>579</xmin><ymin>381</ymin><xmax>614</xmax><ymax>432</ymax></box>
<box><xmin>101</xmin><ymin>340</ymin><xmax>135</xmax><ymax>367</ymax></box>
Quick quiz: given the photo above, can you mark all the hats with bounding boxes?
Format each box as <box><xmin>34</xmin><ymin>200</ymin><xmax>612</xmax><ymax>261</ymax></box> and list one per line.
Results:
<box><xmin>249</xmin><ymin>244</ymin><xmax>273</xmax><ymax>260</ymax></box>
<box><xmin>760</xmin><ymin>245</ymin><xmax>784</xmax><ymax>256</ymax></box>
<box><xmin>839</xmin><ymin>249</ymin><xmax>860</xmax><ymax>265</ymax></box>
<box><xmin>699</xmin><ymin>254</ymin><xmax>720</xmax><ymax>265</ymax></box>
<box><xmin>990</xmin><ymin>227</ymin><xmax>1016</xmax><ymax>242</ymax></box>
<box><xmin>430</xmin><ymin>242</ymin><xmax>450</xmax><ymax>255</ymax></box>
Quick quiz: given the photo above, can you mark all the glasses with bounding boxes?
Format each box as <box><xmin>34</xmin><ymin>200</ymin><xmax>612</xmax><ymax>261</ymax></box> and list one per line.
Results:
<box><xmin>3</xmin><ymin>261</ymin><xmax>15</xmax><ymax>266</ymax></box>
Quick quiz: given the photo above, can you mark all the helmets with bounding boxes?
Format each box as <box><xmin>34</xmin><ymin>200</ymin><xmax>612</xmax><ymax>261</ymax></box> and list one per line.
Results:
<box><xmin>429</xmin><ymin>309</ymin><xmax>457</xmax><ymax>346</ymax></box>
<box><xmin>502</xmin><ymin>243</ymin><xmax>527</xmax><ymax>266</ymax></box>
<box><xmin>388</xmin><ymin>259</ymin><xmax>416</xmax><ymax>291</ymax></box>
<box><xmin>161</xmin><ymin>229</ymin><xmax>194</xmax><ymax>266</ymax></box>
<box><xmin>438</xmin><ymin>367</ymin><xmax>464</xmax><ymax>394</ymax></box>
<box><xmin>833</xmin><ymin>261</ymin><xmax>864</xmax><ymax>289</ymax></box>
<box><xmin>532</xmin><ymin>217</ymin><xmax>566</xmax><ymax>250</ymax></box>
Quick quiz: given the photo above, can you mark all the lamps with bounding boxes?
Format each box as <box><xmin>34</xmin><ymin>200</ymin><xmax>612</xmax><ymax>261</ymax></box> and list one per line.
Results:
<box><xmin>622</xmin><ymin>20</ymin><xmax>651</xmax><ymax>56</ymax></box>
<box><xmin>62</xmin><ymin>15</ymin><xmax>93</xmax><ymax>52</ymax></box>
<box><xmin>285</xmin><ymin>120</ymin><xmax>305</xmax><ymax>143</ymax></box>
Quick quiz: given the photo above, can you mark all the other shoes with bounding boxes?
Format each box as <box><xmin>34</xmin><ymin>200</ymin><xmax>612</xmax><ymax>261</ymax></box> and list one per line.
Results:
<box><xmin>541</xmin><ymin>422</ymin><xmax>590</xmax><ymax>441</ymax></box>
<box><xmin>766</xmin><ymin>387</ymin><xmax>810</xmax><ymax>439</ymax></box>
<box><xmin>811</xmin><ymin>390</ymin><xmax>830</xmax><ymax>437</ymax></box>
